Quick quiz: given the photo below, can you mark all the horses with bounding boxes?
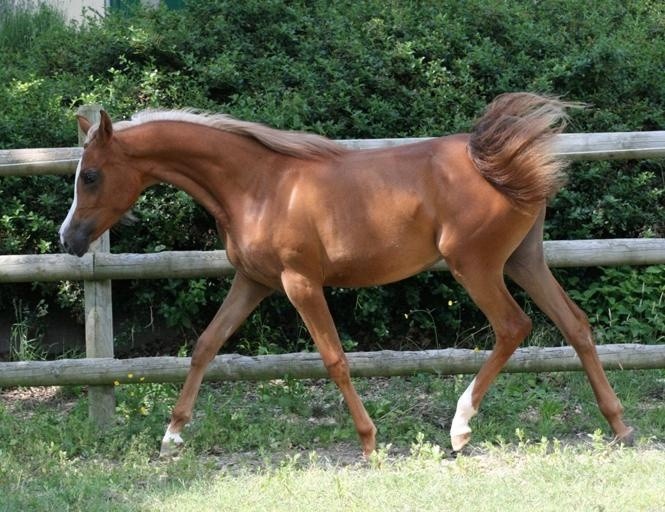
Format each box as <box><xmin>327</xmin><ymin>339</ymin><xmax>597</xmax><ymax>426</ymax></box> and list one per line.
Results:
<box><xmin>58</xmin><ymin>89</ymin><xmax>634</xmax><ymax>465</ymax></box>
